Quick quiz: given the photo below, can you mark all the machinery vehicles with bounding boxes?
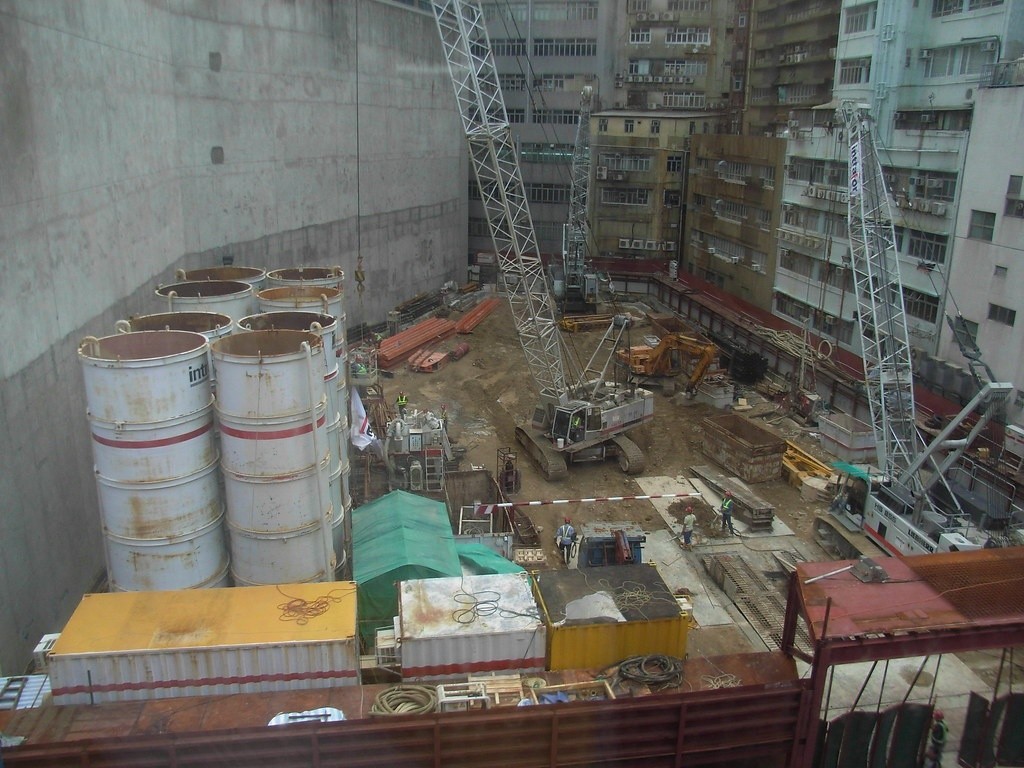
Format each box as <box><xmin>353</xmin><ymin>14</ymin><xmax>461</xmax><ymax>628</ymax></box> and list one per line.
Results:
<box><xmin>616</xmin><ymin>332</ymin><xmax>718</xmax><ymax>407</ymax></box>
<box><xmin>348</xmin><ymin>1</ymin><xmax>655</xmax><ymax>495</ymax></box>
<box><xmin>551</xmin><ymin>86</ymin><xmax>617</xmax><ymax>315</ymax></box>
<box><xmin>776</xmin><ymin>97</ymin><xmax>1018</xmax><ymax>558</ymax></box>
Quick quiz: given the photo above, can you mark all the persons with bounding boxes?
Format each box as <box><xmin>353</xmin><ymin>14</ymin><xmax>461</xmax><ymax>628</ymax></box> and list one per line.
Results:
<box><xmin>394</xmin><ymin>391</ymin><xmax>408</xmax><ymax>421</ymax></box>
<box><xmin>569</xmin><ymin>414</ymin><xmax>582</xmax><ymax>439</ymax></box>
<box><xmin>555</xmin><ymin>517</ymin><xmax>577</xmax><ymax>565</ymax></box>
<box><xmin>829</xmin><ymin>488</ymin><xmax>850</xmax><ymax>515</ymax></box>
<box><xmin>352</xmin><ymin>356</ymin><xmax>367</xmax><ymax>377</ymax></box>
<box><xmin>679</xmin><ymin>507</ymin><xmax>696</xmax><ymax>552</ymax></box>
<box><xmin>929</xmin><ymin>709</ymin><xmax>949</xmax><ymax>768</ymax></box>
<box><xmin>718</xmin><ymin>492</ymin><xmax>734</xmax><ymax>536</ymax></box>
<box><xmin>440</xmin><ymin>405</ymin><xmax>447</xmax><ymax>432</ymax></box>
<box><xmin>373</xmin><ymin>332</ymin><xmax>382</xmax><ymax>348</ymax></box>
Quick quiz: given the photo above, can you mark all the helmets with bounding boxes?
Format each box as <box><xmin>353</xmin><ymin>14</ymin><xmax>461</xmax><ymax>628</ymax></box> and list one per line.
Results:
<box><xmin>685</xmin><ymin>507</ymin><xmax>692</xmax><ymax>513</ymax></box>
<box><xmin>725</xmin><ymin>491</ymin><xmax>731</xmax><ymax>497</ymax></box>
<box><xmin>565</xmin><ymin>517</ymin><xmax>570</xmax><ymax>522</ymax></box>
<box><xmin>399</xmin><ymin>391</ymin><xmax>404</xmax><ymax>394</ymax></box>
<box><xmin>441</xmin><ymin>405</ymin><xmax>446</xmax><ymax>409</ymax></box>
